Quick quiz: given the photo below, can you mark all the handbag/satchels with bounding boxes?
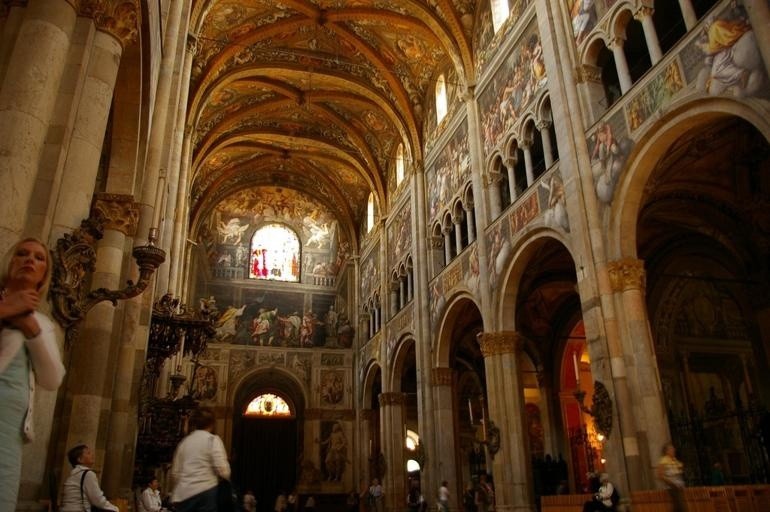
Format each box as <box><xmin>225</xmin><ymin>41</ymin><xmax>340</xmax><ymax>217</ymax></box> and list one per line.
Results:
<box><xmin>217</xmin><ymin>476</ymin><xmax>232</xmax><ymax>512</ymax></box>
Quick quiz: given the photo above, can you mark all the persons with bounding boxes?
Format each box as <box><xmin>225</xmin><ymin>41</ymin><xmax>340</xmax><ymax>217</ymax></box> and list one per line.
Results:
<box><xmin>321</xmin><ymin>371</ymin><xmax>343</xmax><ymax>405</ymax></box>
<box><xmin>1</xmin><ymin>237</ymin><xmax>70</xmax><ymax>510</ymax></box>
<box><xmin>194</xmin><ymin>366</ymin><xmax>217</xmax><ymax>401</ymax></box>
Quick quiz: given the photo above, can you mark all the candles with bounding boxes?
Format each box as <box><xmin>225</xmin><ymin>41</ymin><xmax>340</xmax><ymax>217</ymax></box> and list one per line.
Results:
<box><xmin>572</xmin><ymin>353</ymin><xmax>579</xmax><ymax>379</ymax></box>
<box><xmin>151</xmin><ymin>169</ymin><xmax>166</xmax><ymax>229</ymax></box>
<box><xmin>468</xmin><ymin>399</ymin><xmax>474</xmax><ymax>421</ymax></box>
<box><xmin>174</xmin><ymin>334</ymin><xmax>185</xmax><ymax>375</ymax></box>
